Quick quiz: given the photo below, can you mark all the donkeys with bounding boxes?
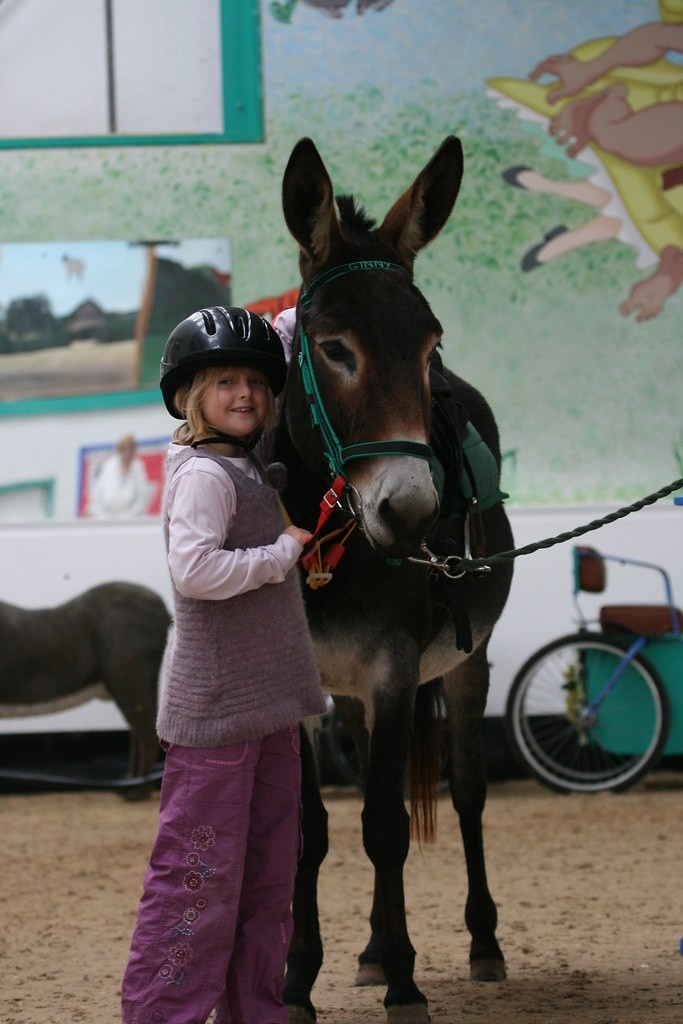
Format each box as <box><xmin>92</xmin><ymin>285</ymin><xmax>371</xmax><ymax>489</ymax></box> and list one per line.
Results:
<box><xmin>267</xmin><ymin>131</ymin><xmax>522</xmax><ymax>1023</ymax></box>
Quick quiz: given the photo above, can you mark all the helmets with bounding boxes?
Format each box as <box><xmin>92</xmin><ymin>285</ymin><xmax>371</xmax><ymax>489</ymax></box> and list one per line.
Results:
<box><xmin>160</xmin><ymin>306</ymin><xmax>287</xmax><ymax>419</ymax></box>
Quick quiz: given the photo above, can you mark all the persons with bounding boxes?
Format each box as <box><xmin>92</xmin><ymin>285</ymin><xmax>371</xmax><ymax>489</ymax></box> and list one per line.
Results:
<box><xmin>121</xmin><ymin>302</ymin><xmax>327</xmax><ymax>1024</ymax></box>
<box><xmin>88</xmin><ymin>434</ymin><xmax>149</xmax><ymax>516</ymax></box>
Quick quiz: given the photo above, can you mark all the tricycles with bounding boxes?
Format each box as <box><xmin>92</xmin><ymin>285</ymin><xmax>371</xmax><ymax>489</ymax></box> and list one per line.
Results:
<box><xmin>503</xmin><ymin>546</ymin><xmax>682</xmax><ymax>794</ymax></box>
